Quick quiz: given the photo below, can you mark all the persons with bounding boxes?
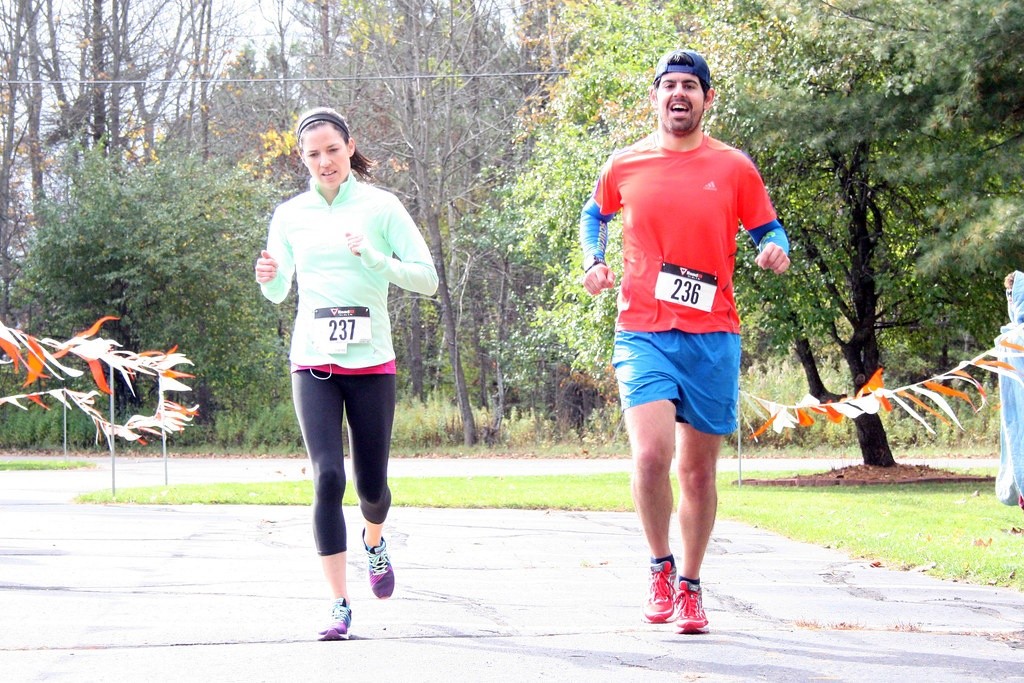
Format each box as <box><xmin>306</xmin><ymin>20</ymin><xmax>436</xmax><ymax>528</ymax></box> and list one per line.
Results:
<box><xmin>994</xmin><ymin>271</ymin><xmax>1024</xmax><ymax>513</ymax></box>
<box><xmin>255</xmin><ymin>107</ymin><xmax>439</xmax><ymax>642</ymax></box>
<box><xmin>580</xmin><ymin>51</ymin><xmax>791</xmax><ymax>635</ymax></box>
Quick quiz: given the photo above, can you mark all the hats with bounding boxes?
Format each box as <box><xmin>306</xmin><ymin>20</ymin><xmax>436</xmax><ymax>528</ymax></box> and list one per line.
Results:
<box><xmin>655</xmin><ymin>47</ymin><xmax>712</xmax><ymax>88</ymax></box>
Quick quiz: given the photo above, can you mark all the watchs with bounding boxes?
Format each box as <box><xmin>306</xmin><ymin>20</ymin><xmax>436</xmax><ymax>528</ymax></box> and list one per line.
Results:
<box><xmin>582</xmin><ymin>255</ymin><xmax>607</xmax><ymax>273</ymax></box>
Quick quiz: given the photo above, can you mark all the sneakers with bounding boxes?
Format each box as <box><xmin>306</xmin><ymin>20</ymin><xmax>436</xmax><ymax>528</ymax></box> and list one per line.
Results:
<box><xmin>674</xmin><ymin>580</ymin><xmax>710</xmax><ymax>635</ymax></box>
<box><xmin>361</xmin><ymin>526</ymin><xmax>395</xmax><ymax>599</ymax></box>
<box><xmin>643</xmin><ymin>561</ymin><xmax>678</xmax><ymax>624</ymax></box>
<box><xmin>316</xmin><ymin>597</ymin><xmax>352</xmax><ymax>640</ymax></box>
